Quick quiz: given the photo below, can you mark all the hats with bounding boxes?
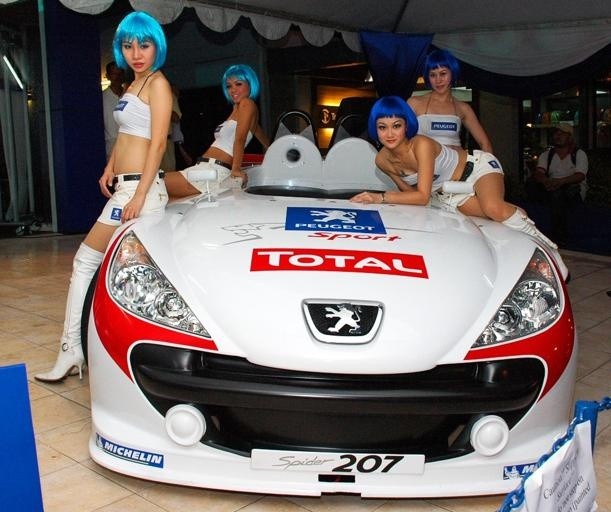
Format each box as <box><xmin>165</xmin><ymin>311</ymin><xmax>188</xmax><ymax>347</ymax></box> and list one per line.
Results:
<box><xmin>550</xmin><ymin>122</ymin><xmax>574</xmax><ymax>135</ymax></box>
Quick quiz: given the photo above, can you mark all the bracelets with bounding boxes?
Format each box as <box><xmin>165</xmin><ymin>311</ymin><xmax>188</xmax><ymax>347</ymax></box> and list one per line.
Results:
<box><xmin>379</xmin><ymin>192</ymin><xmax>385</xmax><ymax>205</ymax></box>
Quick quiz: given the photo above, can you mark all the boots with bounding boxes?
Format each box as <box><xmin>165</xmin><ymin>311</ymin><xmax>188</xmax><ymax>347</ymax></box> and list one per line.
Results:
<box><xmin>500</xmin><ymin>205</ymin><xmax>569</xmax><ymax>282</ymax></box>
<box><xmin>34</xmin><ymin>241</ymin><xmax>105</xmax><ymax>382</ymax></box>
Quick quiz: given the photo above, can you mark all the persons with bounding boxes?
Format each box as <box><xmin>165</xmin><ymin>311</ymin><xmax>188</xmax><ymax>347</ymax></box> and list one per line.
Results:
<box><xmin>32</xmin><ymin>8</ymin><xmax>175</xmax><ymax>384</ymax></box>
<box><xmin>348</xmin><ymin>95</ymin><xmax>571</xmax><ymax>286</ymax></box>
<box><xmin>168</xmin><ymin>82</ymin><xmax>193</xmax><ymax>168</ymax></box>
<box><xmin>517</xmin><ymin>120</ymin><xmax>589</xmax><ymax>235</ymax></box>
<box><xmin>157</xmin><ymin>63</ymin><xmax>272</xmax><ymax>202</ymax></box>
<box><xmin>97</xmin><ymin>59</ymin><xmax>127</xmax><ymax>168</ymax></box>
<box><xmin>402</xmin><ymin>45</ymin><xmax>493</xmax><ymax>156</ymax></box>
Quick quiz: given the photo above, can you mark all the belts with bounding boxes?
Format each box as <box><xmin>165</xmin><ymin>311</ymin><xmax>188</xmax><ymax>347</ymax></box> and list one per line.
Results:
<box><xmin>196</xmin><ymin>156</ymin><xmax>232</xmax><ymax>170</ymax></box>
<box><xmin>437</xmin><ymin>149</ymin><xmax>474</xmax><ymax>195</ymax></box>
<box><xmin>113</xmin><ymin>171</ymin><xmax>165</xmax><ymax>184</ymax></box>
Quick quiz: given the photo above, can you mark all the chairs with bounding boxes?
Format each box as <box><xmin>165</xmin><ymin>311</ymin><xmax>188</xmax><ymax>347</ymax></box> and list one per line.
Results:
<box><xmin>272</xmin><ymin>107</ymin><xmax>317</xmax><ymax>164</ymax></box>
<box><xmin>329</xmin><ymin>111</ymin><xmax>371</xmax><ymax>149</ymax></box>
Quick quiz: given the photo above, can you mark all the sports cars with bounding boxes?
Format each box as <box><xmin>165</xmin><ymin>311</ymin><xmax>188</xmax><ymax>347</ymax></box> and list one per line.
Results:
<box><xmin>85</xmin><ymin>109</ymin><xmax>580</xmax><ymax>502</ymax></box>
